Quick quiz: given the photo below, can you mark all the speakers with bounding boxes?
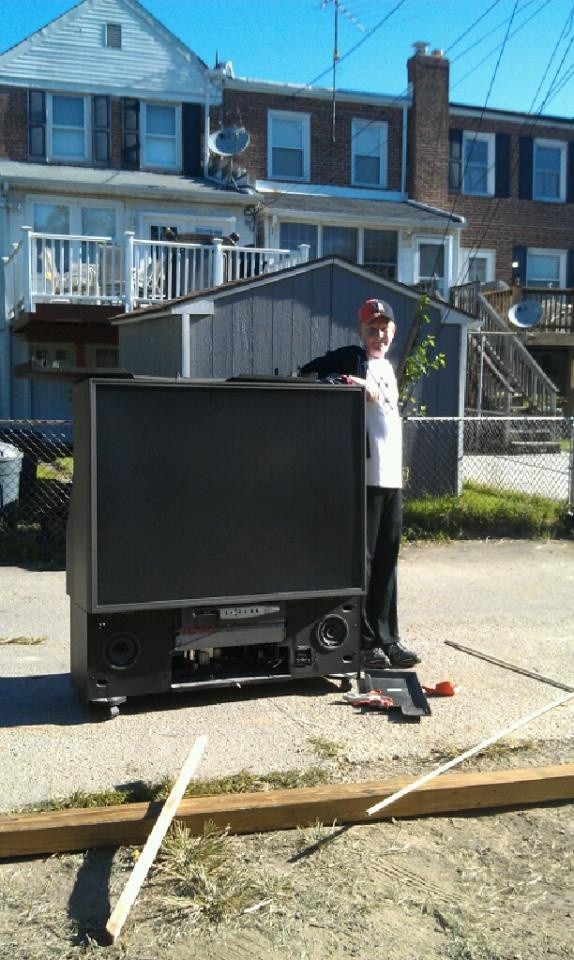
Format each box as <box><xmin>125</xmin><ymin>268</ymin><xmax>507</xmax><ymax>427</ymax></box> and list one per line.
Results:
<box><xmin>64</xmin><ymin>376</ymin><xmax>372</xmax><ymax>705</ymax></box>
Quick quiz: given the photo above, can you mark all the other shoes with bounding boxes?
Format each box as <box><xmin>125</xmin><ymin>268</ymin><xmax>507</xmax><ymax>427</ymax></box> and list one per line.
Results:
<box><xmin>383</xmin><ymin>641</ymin><xmax>421</xmax><ymax>667</ymax></box>
<box><xmin>360</xmin><ymin>647</ymin><xmax>390</xmax><ymax>669</ymax></box>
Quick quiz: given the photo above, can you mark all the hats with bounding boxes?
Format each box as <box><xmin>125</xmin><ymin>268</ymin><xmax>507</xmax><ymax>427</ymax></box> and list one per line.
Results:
<box><xmin>358</xmin><ymin>298</ymin><xmax>394</xmax><ymax>325</ymax></box>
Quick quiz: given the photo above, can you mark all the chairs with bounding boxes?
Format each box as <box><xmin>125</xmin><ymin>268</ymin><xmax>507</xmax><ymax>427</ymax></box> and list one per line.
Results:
<box><xmin>37</xmin><ymin>244</ymin><xmax>165</xmax><ymax>295</ymax></box>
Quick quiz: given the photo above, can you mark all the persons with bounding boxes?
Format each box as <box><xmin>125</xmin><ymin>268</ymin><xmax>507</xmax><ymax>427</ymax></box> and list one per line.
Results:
<box><xmin>338</xmin><ymin>299</ymin><xmax>422</xmax><ymax>668</ymax></box>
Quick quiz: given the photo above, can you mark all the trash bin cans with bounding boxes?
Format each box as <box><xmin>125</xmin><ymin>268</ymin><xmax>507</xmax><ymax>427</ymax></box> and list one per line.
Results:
<box><xmin>0</xmin><ymin>441</ymin><xmax>24</xmax><ymax>536</ymax></box>
